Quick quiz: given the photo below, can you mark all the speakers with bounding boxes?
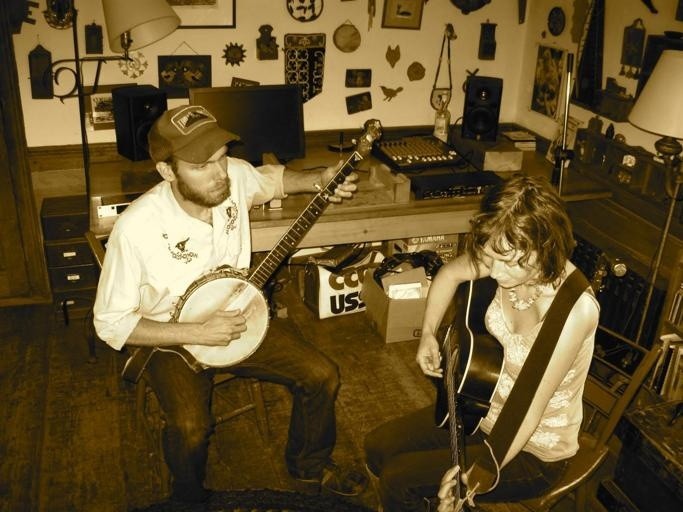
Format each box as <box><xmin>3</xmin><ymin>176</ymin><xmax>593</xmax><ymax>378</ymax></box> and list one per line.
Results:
<box><xmin>461</xmin><ymin>75</ymin><xmax>503</xmax><ymax>142</ymax></box>
<box><xmin>111</xmin><ymin>84</ymin><xmax>168</xmax><ymax>162</ymax></box>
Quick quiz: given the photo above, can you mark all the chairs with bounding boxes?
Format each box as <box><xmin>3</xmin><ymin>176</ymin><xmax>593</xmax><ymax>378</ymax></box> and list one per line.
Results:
<box><xmin>517</xmin><ymin>318</ymin><xmax>666</xmax><ymax>511</ymax></box>
<box><xmin>612</xmin><ymin>316</ymin><xmax>682</xmax><ymax>510</ymax></box>
<box><xmin>78</xmin><ymin>230</ymin><xmax>289</xmax><ymax>500</ymax></box>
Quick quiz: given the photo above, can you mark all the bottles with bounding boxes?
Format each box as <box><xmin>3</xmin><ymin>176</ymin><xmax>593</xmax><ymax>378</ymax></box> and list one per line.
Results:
<box><xmin>433</xmin><ymin>89</ymin><xmax>450</xmax><ymax>143</ymax></box>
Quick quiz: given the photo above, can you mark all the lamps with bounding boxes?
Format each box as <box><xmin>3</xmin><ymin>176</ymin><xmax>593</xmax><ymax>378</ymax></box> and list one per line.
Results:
<box><xmin>627</xmin><ymin>48</ymin><xmax>683</xmax><ymax>202</ymax></box>
<box><xmin>67</xmin><ymin>1</ymin><xmax>181</xmax><ymax>230</ymax></box>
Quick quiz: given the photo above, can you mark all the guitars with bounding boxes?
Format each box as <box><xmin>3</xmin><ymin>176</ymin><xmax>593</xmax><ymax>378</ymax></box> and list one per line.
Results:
<box><xmin>431</xmin><ymin>277</ymin><xmax>505</xmax><ymax>511</ymax></box>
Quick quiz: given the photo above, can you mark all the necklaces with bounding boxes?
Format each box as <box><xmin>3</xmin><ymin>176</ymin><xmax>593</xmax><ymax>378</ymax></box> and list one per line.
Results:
<box><xmin>507</xmin><ymin>287</ymin><xmax>544</xmax><ymax>310</ymax></box>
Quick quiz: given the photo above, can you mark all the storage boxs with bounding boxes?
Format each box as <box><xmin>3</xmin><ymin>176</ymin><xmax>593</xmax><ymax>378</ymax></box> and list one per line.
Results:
<box><xmin>304</xmin><ymin>233</ymin><xmax>462</xmax><ymax>345</ymax></box>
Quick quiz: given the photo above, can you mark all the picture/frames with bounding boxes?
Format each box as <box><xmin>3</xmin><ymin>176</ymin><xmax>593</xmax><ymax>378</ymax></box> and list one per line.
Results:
<box><xmin>529</xmin><ymin>41</ymin><xmax>569</xmax><ymax>120</ymax></box>
<box><xmin>166</xmin><ymin>0</ymin><xmax>236</xmax><ymax>30</ymax></box>
<box><xmin>380</xmin><ymin>0</ymin><xmax>425</xmax><ymax>31</ymax></box>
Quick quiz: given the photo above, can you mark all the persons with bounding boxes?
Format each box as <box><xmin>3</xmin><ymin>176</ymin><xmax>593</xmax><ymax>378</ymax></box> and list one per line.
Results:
<box><xmin>363</xmin><ymin>171</ymin><xmax>601</xmax><ymax>512</ymax></box>
<box><xmin>92</xmin><ymin>105</ymin><xmax>368</xmax><ymax>512</ymax></box>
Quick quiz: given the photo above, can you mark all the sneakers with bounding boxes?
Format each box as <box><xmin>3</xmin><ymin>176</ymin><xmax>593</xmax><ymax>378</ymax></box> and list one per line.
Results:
<box><xmin>289</xmin><ymin>457</ymin><xmax>370</xmax><ymax>496</ymax></box>
<box><xmin>171</xmin><ymin>493</ymin><xmax>207</xmax><ymax>512</ymax></box>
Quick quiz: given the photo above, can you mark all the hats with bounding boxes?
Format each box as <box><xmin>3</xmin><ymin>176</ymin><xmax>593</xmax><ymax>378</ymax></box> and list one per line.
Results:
<box><xmin>146</xmin><ymin>104</ymin><xmax>241</xmax><ymax>164</ymax></box>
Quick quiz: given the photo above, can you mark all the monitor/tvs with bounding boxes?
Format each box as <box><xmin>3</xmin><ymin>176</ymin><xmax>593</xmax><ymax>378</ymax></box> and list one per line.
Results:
<box><xmin>187</xmin><ymin>85</ymin><xmax>306</xmax><ymax>171</ymax></box>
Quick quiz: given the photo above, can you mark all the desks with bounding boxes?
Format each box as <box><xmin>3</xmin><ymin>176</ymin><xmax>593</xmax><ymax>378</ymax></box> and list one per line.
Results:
<box><xmin>83</xmin><ymin>159</ymin><xmax>501</xmax><ymax>252</ymax></box>
